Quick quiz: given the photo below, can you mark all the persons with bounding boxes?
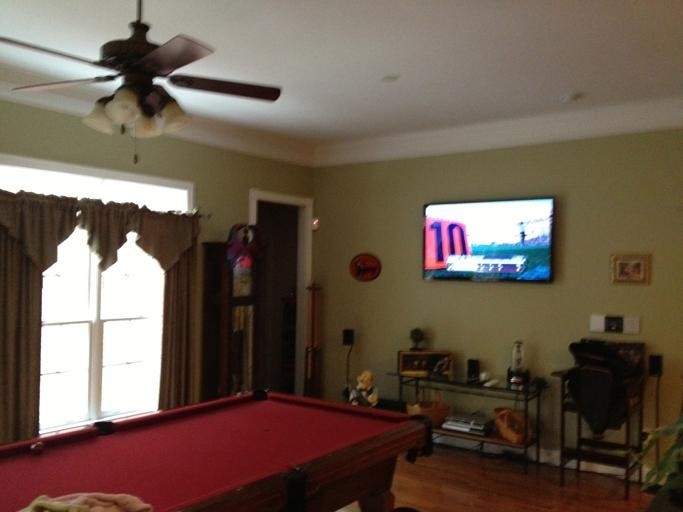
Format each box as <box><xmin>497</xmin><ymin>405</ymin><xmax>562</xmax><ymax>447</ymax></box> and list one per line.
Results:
<box><xmin>519</xmin><ymin>221</ymin><xmax>528</xmax><ymax>247</ymax></box>
<box><xmin>349</xmin><ymin>370</ymin><xmax>380</xmax><ymax>409</ymax></box>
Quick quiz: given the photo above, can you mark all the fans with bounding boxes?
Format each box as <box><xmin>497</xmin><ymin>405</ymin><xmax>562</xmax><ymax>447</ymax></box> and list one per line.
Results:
<box><xmin>0</xmin><ymin>1</ymin><xmax>286</xmax><ymax>108</ymax></box>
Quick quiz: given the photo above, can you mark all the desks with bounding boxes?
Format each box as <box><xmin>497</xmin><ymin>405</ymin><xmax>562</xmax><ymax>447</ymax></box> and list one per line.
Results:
<box><xmin>0</xmin><ymin>381</ymin><xmax>435</xmax><ymax>512</ymax></box>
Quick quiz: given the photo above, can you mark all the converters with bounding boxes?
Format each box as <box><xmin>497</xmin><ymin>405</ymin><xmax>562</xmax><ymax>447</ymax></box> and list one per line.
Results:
<box><xmin>343</xmin><ymin>330</ymin><xmax>354</xmax><ymax>344</ymax></box>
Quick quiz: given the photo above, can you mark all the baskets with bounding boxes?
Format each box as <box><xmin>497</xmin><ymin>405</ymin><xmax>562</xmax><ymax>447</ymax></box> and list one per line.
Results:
<box><xmin>404</xmin><ymin>391</ymin><xmax>450</xmax><ymax>427</ymax></box>
<box><xmin>495</xmin><ymin>405</ymin><xmax>533</xmax><ymax>444</ymax></box>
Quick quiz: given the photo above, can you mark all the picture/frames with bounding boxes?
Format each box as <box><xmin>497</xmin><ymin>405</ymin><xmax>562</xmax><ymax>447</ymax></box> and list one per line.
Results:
<box><xmin>608</xmin><ymin>250</ymin><xmax>653</xmax><ymax>287</ymax></box>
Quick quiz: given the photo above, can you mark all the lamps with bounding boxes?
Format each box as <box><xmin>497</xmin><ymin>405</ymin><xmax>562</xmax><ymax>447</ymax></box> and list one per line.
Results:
<box><xmin>77</xmin><ymin>81</ymin><xmax>189</xmax><ymax>167</ymax></box>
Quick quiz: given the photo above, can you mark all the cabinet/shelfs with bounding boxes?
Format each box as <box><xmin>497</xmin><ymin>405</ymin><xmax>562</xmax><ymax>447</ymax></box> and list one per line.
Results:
<box><xmin>382</xmin><ymin>365</ymin><xmax>552</xmax><ymax>475</ymax></box>
<box><xmin>198</xmin><ymin>237</ymin><xmax>270</xmax><ymax>405</ymax></box>
<box><xmin>549</xmin><ymin>358</ymin><xmax>651</xmax><ymax>502</ymax></box>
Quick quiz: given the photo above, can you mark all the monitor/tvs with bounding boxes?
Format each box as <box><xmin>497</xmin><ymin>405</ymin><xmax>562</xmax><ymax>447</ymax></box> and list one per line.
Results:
<box><xmin>422</xmin><ymin>196</ymin><xmax>555</xmax><ymax>283</ymax></box>
